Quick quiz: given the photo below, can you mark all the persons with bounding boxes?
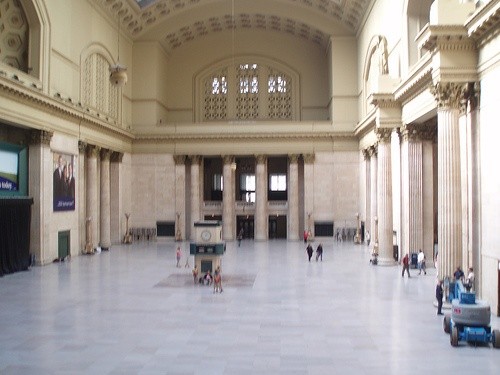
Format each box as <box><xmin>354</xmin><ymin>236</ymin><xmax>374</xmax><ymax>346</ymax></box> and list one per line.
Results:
<box><xmin>184</xmin><ymin>257</ymin><xmax>190</xmax><ymax>268</ymax></box>
<box><xmin>401</xmin><ymin>254</ymin><xmax>411</xmax><ymax>278</ymax></box>
<box><xmin>417</xmin><ymin>250</ymin><xmax>426</xmax><ymax>275</ymax></box>
<box><xmin>316</xmin><ymin>244</ymin><xmax>323</xmax><ymax>261</ymax></box>
<box><xmin>98</xmin><ymin>240</ymin><xmax>110</xmax><ymax>252</ymax></box>
<box><xmin>128</xmin><ymin>227</ymin><xmax>157</xmax><ymax>243</ymax></box>
<box><xmin>335</xmin><ymin>227</ymin><xmax>358</xmax><ymax>243</ymax></box>
<box><xmin>54</xmin><ymin>156</ymin><xmax>75</xmax><ymax>200</ymax></box>
<box><xmin>193</xmin><ymin>265</ymin><xmax>223</xmax><ymax>293</ymax></box>
<box><xmin>303</xmin><ymin>231</ymin><xmax>308</xmax><ymax>243</ymax></box>
<box><xmin>307</xmin><ymin>244</ymin><xmax>313</xmax><ymax>261</ymax></box>
<box><xmin>366</xmin><ymin>230</ymin><xmax>371</xmax><ymax>246</ymax></box>
<box><xmin>177</xmin><ymin>247</ymin><xmax>181</xmax><ymax>267</ymax></box>
<box><xmin>238</xmin><ymin>230</ymin><xmax>243</xmax><ymax>246</ymax></box>
<box><xmin>436</xmin><ymin>266</ymin><xmax>476</xmax><ymax>315</ymax></box>
